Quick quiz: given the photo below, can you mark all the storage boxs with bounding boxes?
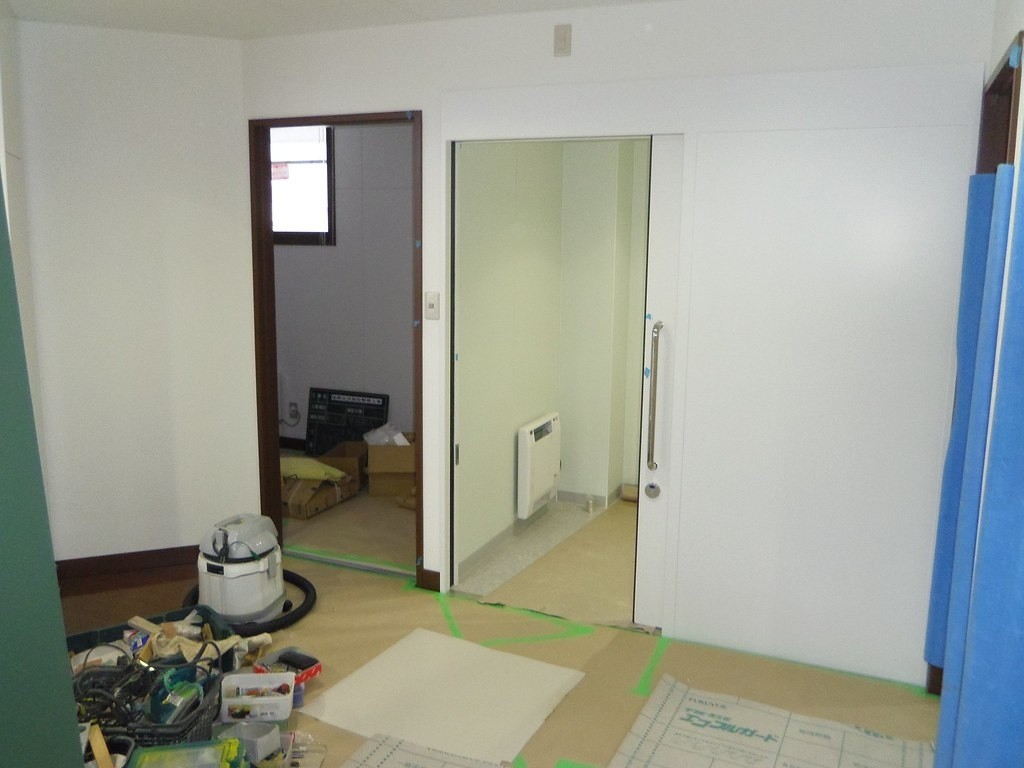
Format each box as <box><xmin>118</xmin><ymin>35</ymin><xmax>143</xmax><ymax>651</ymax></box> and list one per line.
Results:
<box><xmin>364</xmin><ymin>422</ymin><xmax>418</xmax><ymax>499</ymax></box>
<box><xmin>279</xmin><ymin>454</ymin><xmax>362</xmax><ymax>520</ymax></box>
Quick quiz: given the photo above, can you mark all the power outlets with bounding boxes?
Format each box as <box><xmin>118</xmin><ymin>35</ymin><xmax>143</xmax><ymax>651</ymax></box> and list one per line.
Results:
<box><xmin>288</xmin><ymin>402</ymin><xmax>299</xmax><ymax>419</ymax></box>
